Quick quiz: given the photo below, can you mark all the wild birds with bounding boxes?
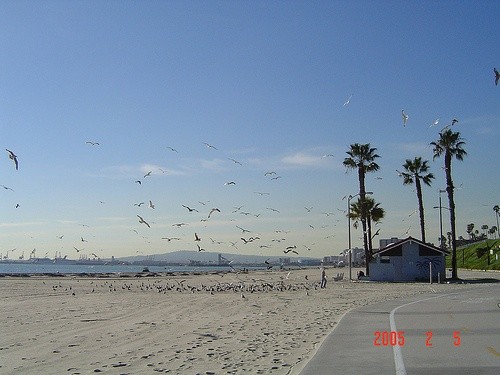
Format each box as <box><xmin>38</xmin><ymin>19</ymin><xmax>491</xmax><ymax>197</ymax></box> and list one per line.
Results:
<box><xmin>445</xmin><ymin>281</ymin><xmax>450</xmax><ymax>284</ymax></box>
<box><xmin>1</xmin><ymin>140</ymin><xmax>351</xmax><ymax>298</ymax></box>
<box><xmin>342</xmin><ymin>94</ymin><xmax>354</xmax><ymax>107</ymax></box>
<box><xmin>460</xmin><ymin>281</ymin><xmax>466</xmax><ymax>284</ymax></box>
<box><xmin>401</xmin><ymin>109</ymin><xmax>409</xmax><ymax>128</ymax></box>
<box><xmin>358</xmin><ymin>165</ymin><xmax>464</xmax><ymax>250</ymax></box>
<box><xmin>429</xmin><ymin>116</ymin><xmax>459</xmax><ymax>133</ymax></box>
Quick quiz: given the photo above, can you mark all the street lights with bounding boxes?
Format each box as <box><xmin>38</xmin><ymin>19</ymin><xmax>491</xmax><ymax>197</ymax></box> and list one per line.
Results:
<box><xmin>439</xmin><ymin>189</ymin><xmax>447</xmax><ymax>244</ymax></box>
<box><xmin>347</xmin><ymin>191</ymin><xmax>374</xmax><ymax>280</ymax></box>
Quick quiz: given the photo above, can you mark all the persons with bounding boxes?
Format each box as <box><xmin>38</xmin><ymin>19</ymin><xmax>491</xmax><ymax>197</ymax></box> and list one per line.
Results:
<box><xmin>321</xmin><ymin>267</ymin><xmax>327</xmax><ymax>288</ymax></box>
